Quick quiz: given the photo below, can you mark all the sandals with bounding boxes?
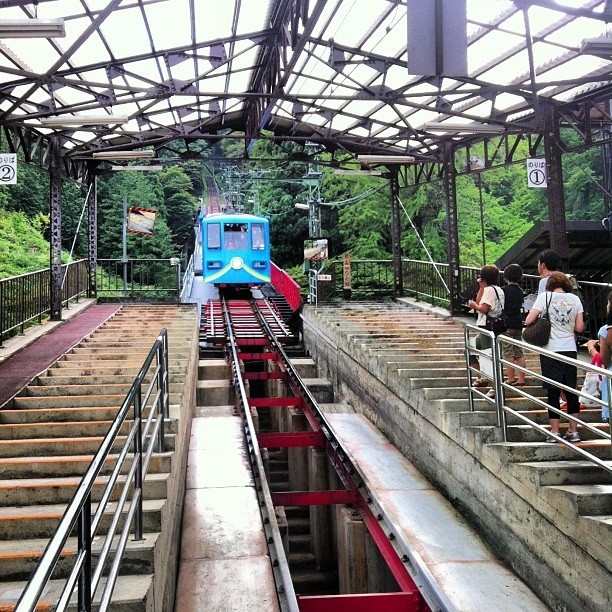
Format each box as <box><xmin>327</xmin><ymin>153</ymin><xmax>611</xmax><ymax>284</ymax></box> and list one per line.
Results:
<box><xmin>486</xmin><ymin>389</ymin><xmax>496</xmax><ymax>398</ymax></box>
<box><xmin>472</xmin><ymin>380</ymin><xmax>488</xmax><ymax>387</ymax></box>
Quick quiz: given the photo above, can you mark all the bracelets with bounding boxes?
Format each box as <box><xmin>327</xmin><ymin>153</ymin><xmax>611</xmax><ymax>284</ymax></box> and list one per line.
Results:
<box><xmin>589</xmin><ymin>347</ymin><xmax>594</xmax><ymax>354</ymax></box>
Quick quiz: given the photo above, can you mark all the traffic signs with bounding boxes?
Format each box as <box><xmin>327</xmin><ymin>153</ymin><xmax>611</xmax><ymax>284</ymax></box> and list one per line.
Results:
<box><xmin>527</xmin><ymin>158</ymin><xmax>549</xmax><ymax>189</ymax></box>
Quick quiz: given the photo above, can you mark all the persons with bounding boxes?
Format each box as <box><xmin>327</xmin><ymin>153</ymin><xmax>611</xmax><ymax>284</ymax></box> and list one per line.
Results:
<box><xmin>468</xmin><ymin>265</ymin><xmax>505</xmax><ymax>399</ymax></box>
<box><xmin>526</xmin><ymin>272</ymin><xmax>584</xmax><ymax>442</ymax></box>
<box><xmin>527</xmin><ymin>250</ymin><xmax>586</xmax><ymax>410</ymax></box>
<box><xmin>587</xmin><ymin>292</ymin><xmax>612</xmax><ymax>431</ymax></box>
<box><xmin>501</xmin><ymin>264</ymin><xmax>527</xmax><ymax>386</ymax></box>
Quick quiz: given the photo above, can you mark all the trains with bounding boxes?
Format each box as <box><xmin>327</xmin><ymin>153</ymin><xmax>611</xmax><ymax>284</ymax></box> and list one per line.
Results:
<box><xmin>201</xmin><ymin>212</ymin><xmax>271</xmax><ymax>294</ymax></box>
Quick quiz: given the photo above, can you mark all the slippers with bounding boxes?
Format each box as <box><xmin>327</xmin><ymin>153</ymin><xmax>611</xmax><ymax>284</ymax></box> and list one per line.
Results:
<box><xmin>511</xmin><ymin>382</ymin><xmax>527</xmax><ymax>385</ymax></box>
<box><xmin>506</xmin><ymin>381</ymin><xmax>515</xmax><ymax>384</ymax></box>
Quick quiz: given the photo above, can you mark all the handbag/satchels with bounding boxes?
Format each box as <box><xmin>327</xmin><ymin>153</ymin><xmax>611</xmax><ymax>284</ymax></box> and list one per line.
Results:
<box><xmin>523</xmin><ymin>292</ymin><xmax>552</xmax><ymax>345</ymax></box>
<box><xmin>578</xmin><ymin>353</ymin><xmax>602</xmax><ymax>407</ymax></box>
<box><xmin>486</xmin><ymin>286</ymin><xmax>508</xmax><ymax>334</ymax></box>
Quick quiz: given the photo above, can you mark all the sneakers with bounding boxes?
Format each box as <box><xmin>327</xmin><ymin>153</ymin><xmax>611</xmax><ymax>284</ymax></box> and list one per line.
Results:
<box><xmin>546</xmin><ymin>433</ymin><xmax>561</xmax><ymax>443</ymax></box>
<box><xmin>545</xmin><ymin>397</ymin><xmax>567</xmax><ymax>410</ymax></box>
<box><xmin>562</xmin><ymin>431</ymin><xmax>580</xmax><ymax>441</ymax></box>
